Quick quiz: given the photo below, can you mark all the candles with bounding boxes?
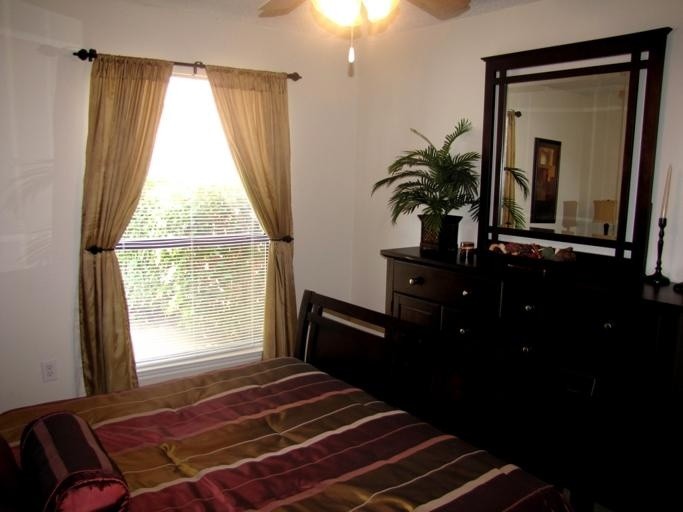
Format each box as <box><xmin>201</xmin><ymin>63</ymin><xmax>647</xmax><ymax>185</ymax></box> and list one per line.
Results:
<box><xmin>661</xmin><ymin>165</ymin><xmax>674</xmax><ymax>219</ymax></box>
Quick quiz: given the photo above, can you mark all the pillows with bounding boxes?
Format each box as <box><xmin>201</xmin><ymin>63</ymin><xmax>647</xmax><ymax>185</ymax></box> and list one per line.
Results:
<box><xmin>20</xmin><ymin>411</ymin><xmax>132</xmax><ymax>512</ymax></box>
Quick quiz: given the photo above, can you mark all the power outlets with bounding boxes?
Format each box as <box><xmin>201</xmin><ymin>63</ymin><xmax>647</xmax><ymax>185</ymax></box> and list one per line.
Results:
<box><xmin>41</xmin><ymin>361</ymin><xmax>58</xmax><ymax>382</ymax></box>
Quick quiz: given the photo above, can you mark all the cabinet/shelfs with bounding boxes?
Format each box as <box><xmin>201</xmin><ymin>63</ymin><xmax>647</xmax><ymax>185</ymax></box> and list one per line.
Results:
<box><xmin>380</xmin><ymin>248</ymin><xmax>682</xmax><ymax>511</ymax></box>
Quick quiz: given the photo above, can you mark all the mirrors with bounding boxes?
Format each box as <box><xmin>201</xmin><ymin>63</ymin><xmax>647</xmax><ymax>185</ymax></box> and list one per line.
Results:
<box><xmin>494</xmin><ymin>61</ymin><xmax>638</xmax><ymax>246</ymax></box>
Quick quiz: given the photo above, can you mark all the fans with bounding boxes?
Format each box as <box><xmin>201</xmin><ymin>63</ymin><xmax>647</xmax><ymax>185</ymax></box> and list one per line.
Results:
<box><xmin>259</xmin><ymin>0</ymin><xmax>469</xmax><ymax>21</ymax></box>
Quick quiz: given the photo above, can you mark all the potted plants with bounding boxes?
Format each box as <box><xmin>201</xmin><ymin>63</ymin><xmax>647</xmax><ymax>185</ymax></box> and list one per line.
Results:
<box><xmin>370</xmin><ymin>118</ymin><xmax>482</xmax><ymax>252</ymax></box>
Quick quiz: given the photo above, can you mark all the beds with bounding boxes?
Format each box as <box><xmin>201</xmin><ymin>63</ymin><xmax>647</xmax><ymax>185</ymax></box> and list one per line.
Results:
<box><xmin>0</xmin><ymin>291</ymin><xmax>576</xmax><ymax>512</ymax></box>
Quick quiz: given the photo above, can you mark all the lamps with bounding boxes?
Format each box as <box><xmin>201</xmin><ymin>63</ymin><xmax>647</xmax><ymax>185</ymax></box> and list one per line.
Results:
<box><xmin>311</xmin><ymin>1</ymin><xmax>396</xmax><ymax>65</ymax></box>
<box><xmin>592</xmin><ymin>200</ymin><xmax>616</xmax><ymax>236</ymax></box>
